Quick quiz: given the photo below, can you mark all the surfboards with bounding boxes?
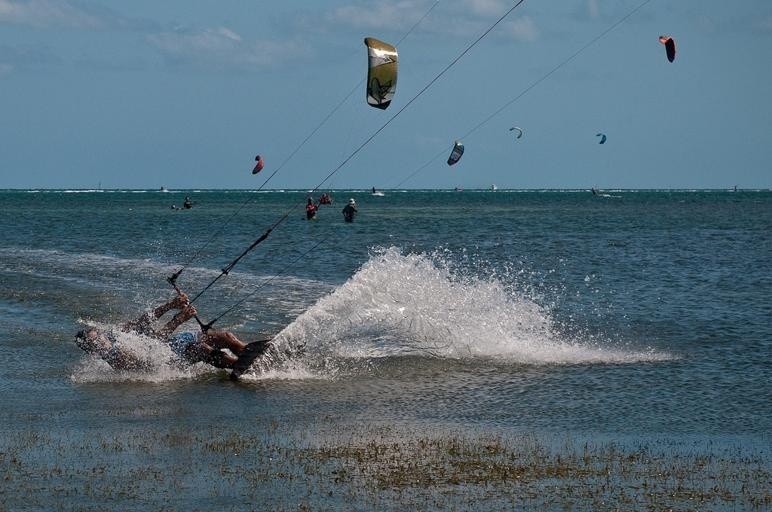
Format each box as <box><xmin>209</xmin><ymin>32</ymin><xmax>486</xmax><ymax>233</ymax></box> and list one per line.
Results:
<box><xmin>226</xmin><ymin>340</ymin><xmax>270</xmax><ymax>379</ymax></box>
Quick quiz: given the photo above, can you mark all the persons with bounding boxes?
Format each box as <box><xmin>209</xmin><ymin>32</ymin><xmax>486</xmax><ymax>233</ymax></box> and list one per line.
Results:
<box><xmin>73</xmin><ymin>289</ymin><xmax>264</xmax><ymax>379</ymax></box>
<box><xmin>318</xmin><ymin>193</ymin><xmax>332</xmax><ymax>204</ymax></box>
<box><xmin>342</xmin><ymin>197</ymin><xmax>359</xmax><ymax>224</ymax></box>
<box><xmin>305</xmin><ymin>195</ymin><xmax>319</xmax><ymax>221</ymax></box>
<box><xmin>182</xmin><ymin>194</ymin><xmax>194</xmax><ymax>210</ymax></box>
